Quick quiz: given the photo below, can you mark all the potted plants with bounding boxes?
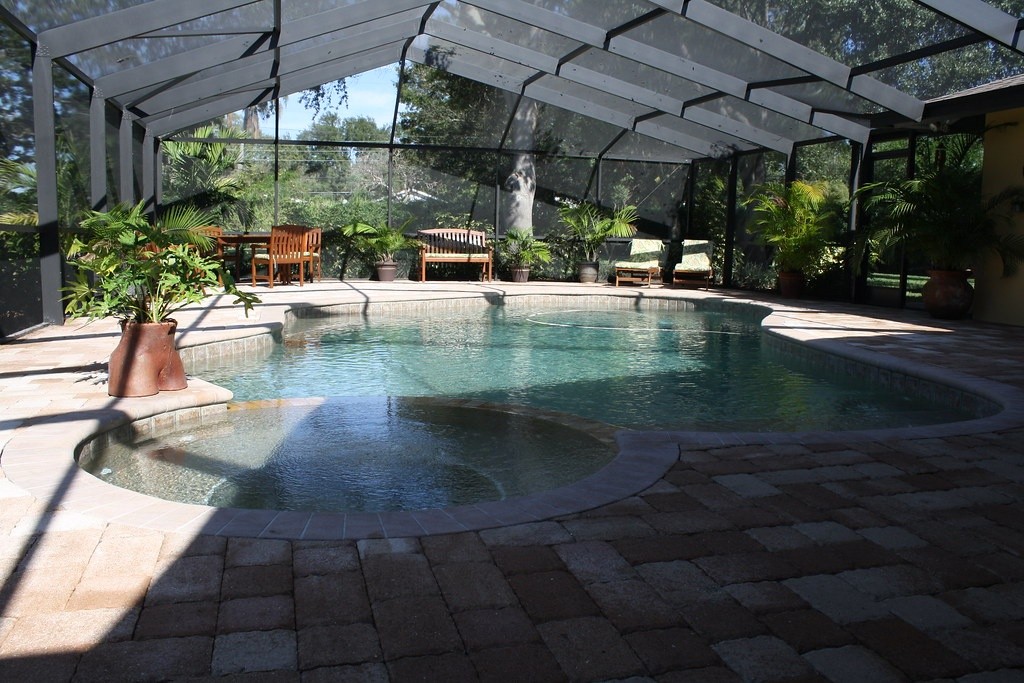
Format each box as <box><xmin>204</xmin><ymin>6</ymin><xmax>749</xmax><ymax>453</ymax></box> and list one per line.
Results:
<box><xmin>343</xmin><ymin>215</ymin><xmax>419</xmax><ymax>281</ymax></box>
<box><xmin>850</xmin><ymin>119</ymin><xmax>1024</xmax><ymax>319</ymax></box>
<box><xmin>739</xmin><ymin>180</ymin><xmax>870</xmax><ymax>304</ymax></box>
<box><xmin>558</xmin><ymin>199</ymin><xmax>638</xmax><ymax>283</ymax></box>
<box><xmin>491</xmin><ymin>225</ymin><xmax>552</xmax><ymax>282</ymax></box>
<box><xmin>66</xmin><ymin>198</ymin><xmax>260</xmax><ymax>398</ymax></box>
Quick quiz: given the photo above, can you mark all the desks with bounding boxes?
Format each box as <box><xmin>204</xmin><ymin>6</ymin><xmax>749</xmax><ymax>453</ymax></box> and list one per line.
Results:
<box><xmin>218</xmin><ymin>231</ymin><xmax>292</xmax><ymax>282</ymax></box>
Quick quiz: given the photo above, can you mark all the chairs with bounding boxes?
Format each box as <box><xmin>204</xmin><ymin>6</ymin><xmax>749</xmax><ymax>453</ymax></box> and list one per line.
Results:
<box><xmin>615</xmin><ymin>239</ymin><xmax>665</xmax><ymax>288</ymax></box>
<box><xmin>251</xmin><ymin>225</ymin><xmax>324</xmax><ymax>289</ymax></box>
<box><xmin>142</xmin><ymin>223</ymin><xmax>223</xmax><ymax>302</ymax></box>
<box><xmin>673</xmin><ymin>239</ymin><xmax>719</xmax><ymax>289</ymax></box>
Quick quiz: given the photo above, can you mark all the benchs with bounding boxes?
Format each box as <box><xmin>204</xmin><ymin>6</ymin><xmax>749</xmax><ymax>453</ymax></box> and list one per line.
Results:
<box><xmin>416</xmin><ymin>228</ymin><xmax>494</xmax><ymax>282</ymax></box>
